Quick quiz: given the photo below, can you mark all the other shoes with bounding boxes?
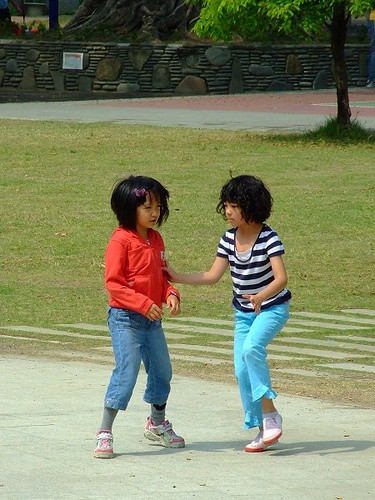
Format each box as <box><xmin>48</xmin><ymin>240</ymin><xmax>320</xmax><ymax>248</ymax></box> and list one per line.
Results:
<box><xmin>244</xmin><ymin>431</ymin><xmax>278</xmax><ymax>451</ymax></box>
<box><xmin>263</xmin><ymin>411</ymin><xmax>283</xmax><ymax>445</ymax></box>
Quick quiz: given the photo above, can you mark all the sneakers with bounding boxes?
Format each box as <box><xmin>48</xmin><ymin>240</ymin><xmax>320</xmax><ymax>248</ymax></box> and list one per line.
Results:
<box><xmin>143</xmin><ymin>417</ymin><xmax>185</xmax><ymax>449</ymax></box>
<box><xmin>94</xmin><ymin>430</ymin><xmax>114</xmax><ymax>459</ymax></box>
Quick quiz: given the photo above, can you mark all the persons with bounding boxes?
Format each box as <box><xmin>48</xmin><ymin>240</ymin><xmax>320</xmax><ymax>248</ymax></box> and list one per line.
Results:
<box><xmin>159</xmin><ymin>170</ymin><xmax>295</xmax><ymax>453</ymax></box>
<box><xmin>92</xmin><ymin>171</ymin><xmax>186</xmax><ymax>459</ymax></box>
